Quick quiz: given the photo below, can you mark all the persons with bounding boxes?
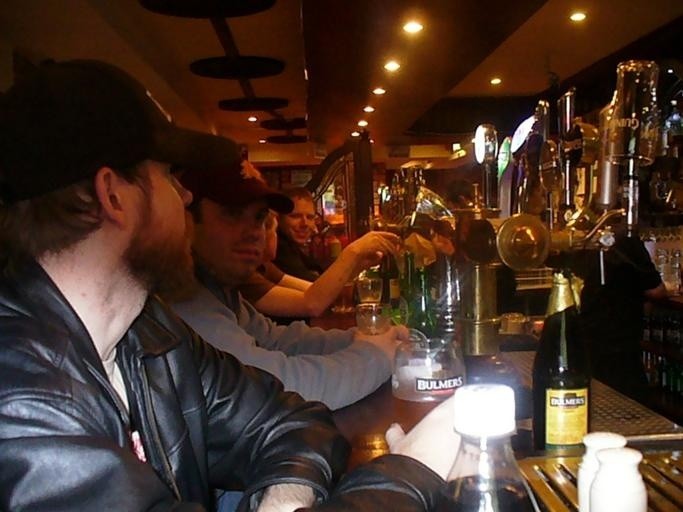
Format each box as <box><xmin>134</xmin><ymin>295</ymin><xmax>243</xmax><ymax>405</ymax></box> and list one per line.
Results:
<box><xmin>0</xmin><ymin>55</ymin><xmax>465</xmax><ymax>512</ymax></box>
<box><xmin>571</xmin><ymin>210</ymin><xmax>669</xmax><ymax>410</ymax></box>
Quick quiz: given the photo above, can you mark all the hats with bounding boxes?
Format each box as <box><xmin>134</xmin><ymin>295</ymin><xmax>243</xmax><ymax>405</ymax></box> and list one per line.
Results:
<box><xmin>180</xmin><ymin>156</ymin><xmax>295</xmax><ymax>215</ymax></box>
<box><xmin>5</xmin><ymin>61</ymin><xmax>234</xmax><ymax>203</ymax></box>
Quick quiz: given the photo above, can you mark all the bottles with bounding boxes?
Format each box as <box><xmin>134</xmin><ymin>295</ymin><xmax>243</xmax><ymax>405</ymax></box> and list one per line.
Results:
<box><xmin>333</xmin><ymin>247</ymin><xmax>464</xmax><ymax>401</ymax></box>
<box><xmin>570</xmin><ymin>431</ymin><xmax>648</xmax><ymax>511</ymax></box>
<box><xmin>654</xmin><ymin>247</ymin><xmax>682</xmax><ymax>289</ymax></box>
<box><xmin>638</xmin><ymin>306</ymin><xmax>683</xmax><ymax>397</ymax></box>
<box><xmin>530</xmin><ymin>267</ymin><xmax>591</xmax><ymax>451</ymax></box>
<box><xmin>428</xmin><ymin>382</ymin><xmax>534</xmax><ymax>512</ymax></box>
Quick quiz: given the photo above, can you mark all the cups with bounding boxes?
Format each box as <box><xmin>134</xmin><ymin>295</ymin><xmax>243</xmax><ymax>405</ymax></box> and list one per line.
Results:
<box><xmin>601</xmin><ymin>59</ymin><xmax>660</xmax><ymax>168</ymax></box>
